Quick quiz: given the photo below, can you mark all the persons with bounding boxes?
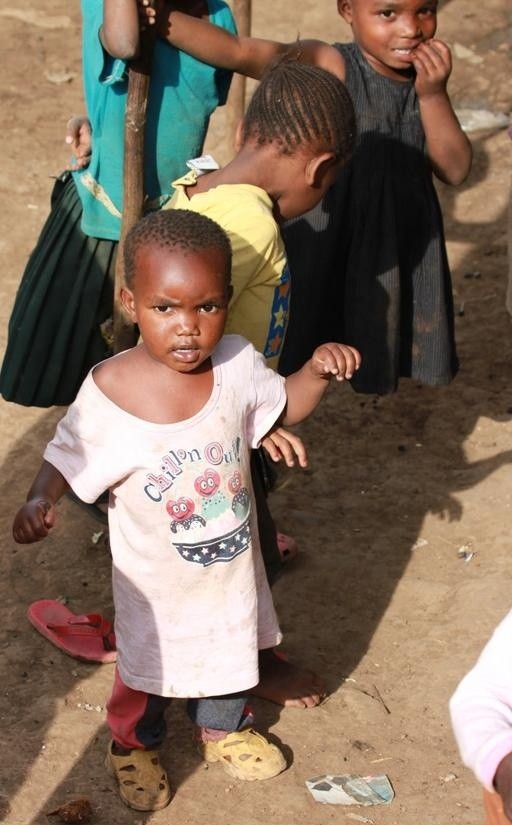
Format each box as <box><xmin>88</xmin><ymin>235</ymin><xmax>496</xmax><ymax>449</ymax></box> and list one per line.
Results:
<box><xmin>13</xmin><ymin>208</ymin><xmax>361</xmax><ymax>812</ymax></box>
<box><xmin>161</xmin><ymin>55</ymin><xmax>360</xmax><ymax>710</ymax></box>
<box><xmin>1</xmin><ymin>1</ymin><xmax>250</xmax><ymax>407</ymax></box>
<box><xmin>452</xmin><ymin>611</ymin><xmax>510</xmax><ymax>817</ymax></box>
<box><xmin>138</xmin><ymin>2</ymin><xmax>474</xmax><ymax>393</ymax></box>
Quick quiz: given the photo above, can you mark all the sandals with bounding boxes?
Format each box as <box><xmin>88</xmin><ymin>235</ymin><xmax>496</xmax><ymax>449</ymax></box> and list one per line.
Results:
<box><xmin>195</xmin><ymin>730</ymin><xmax>287</xmax><ymax>781</ymax></box>
<box><xmin>106</xmin><ymin>738</ymin><xmax>170</xmax><ymax>811</ymax></box>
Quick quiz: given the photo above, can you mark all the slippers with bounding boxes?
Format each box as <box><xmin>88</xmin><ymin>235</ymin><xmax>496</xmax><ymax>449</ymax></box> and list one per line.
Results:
<box><xmin>28</xmin><ymin>599</ymin><xmax>119</xmax><ymax>662</ymax></box>
